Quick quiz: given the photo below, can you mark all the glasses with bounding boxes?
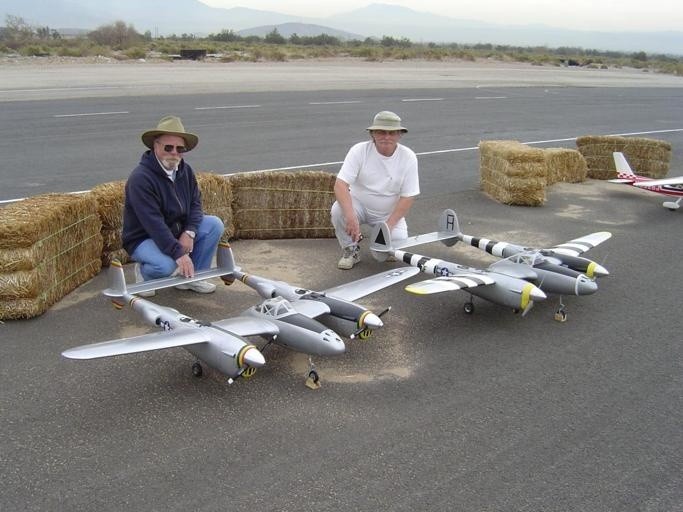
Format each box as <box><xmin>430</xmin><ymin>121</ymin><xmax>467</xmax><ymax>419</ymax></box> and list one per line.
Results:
<box><xmin>154</xmin><ymin>140</ymin><xmax>188</xmax><ymax>154</ymax></box>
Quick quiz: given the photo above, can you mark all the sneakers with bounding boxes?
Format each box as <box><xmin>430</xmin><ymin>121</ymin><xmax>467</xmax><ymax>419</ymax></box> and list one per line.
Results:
<box><xmin>336</xmin><ymin>245</ymin><xmax>360</xmax><ymax>270</ymax></box>
<box><xmin>174</xmin><ymin>280</ymin><xmax>216</xmax><ymax>294</ymax></box>
<box><xmin>133</xmin><ymin>262</ymin><xmax>156</xmax><ymax>298</ymax></box>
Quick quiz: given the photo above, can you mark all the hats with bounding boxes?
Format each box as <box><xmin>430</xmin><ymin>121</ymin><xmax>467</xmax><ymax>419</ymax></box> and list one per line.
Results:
<box><xmin>140</xmin><ymin>115</ymin><xmax>199</xmax><ymax>153</ymax></box>
<box><xmin>365</xmin><ymin>110</ymin><xmax>409</xmax><ymax>134</ymax></box>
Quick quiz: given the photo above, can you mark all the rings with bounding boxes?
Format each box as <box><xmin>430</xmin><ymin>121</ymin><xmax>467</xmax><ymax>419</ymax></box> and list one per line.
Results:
<box><xmin>188</xmin><ymin>251</ymin><xmax>192</xmax><ymax>253</ymax></box>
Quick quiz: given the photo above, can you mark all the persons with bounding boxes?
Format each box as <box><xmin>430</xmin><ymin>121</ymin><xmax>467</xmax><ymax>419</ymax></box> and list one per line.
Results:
<box><xmin>118</xmin><ymin>115</ymin><xmax>226</xmax><ymax>297</ymax></box>
<box><xmin>328</xmin><ymin>110</ymin><xmax>422</xmax><ymax>272</ymax></box>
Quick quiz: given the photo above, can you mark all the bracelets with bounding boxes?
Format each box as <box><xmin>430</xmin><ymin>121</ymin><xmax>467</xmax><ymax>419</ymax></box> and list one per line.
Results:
<box><xmin>184</xmin><ymin>231</ymin><xmax>195</xmax><ymax>239</ymax></box>
